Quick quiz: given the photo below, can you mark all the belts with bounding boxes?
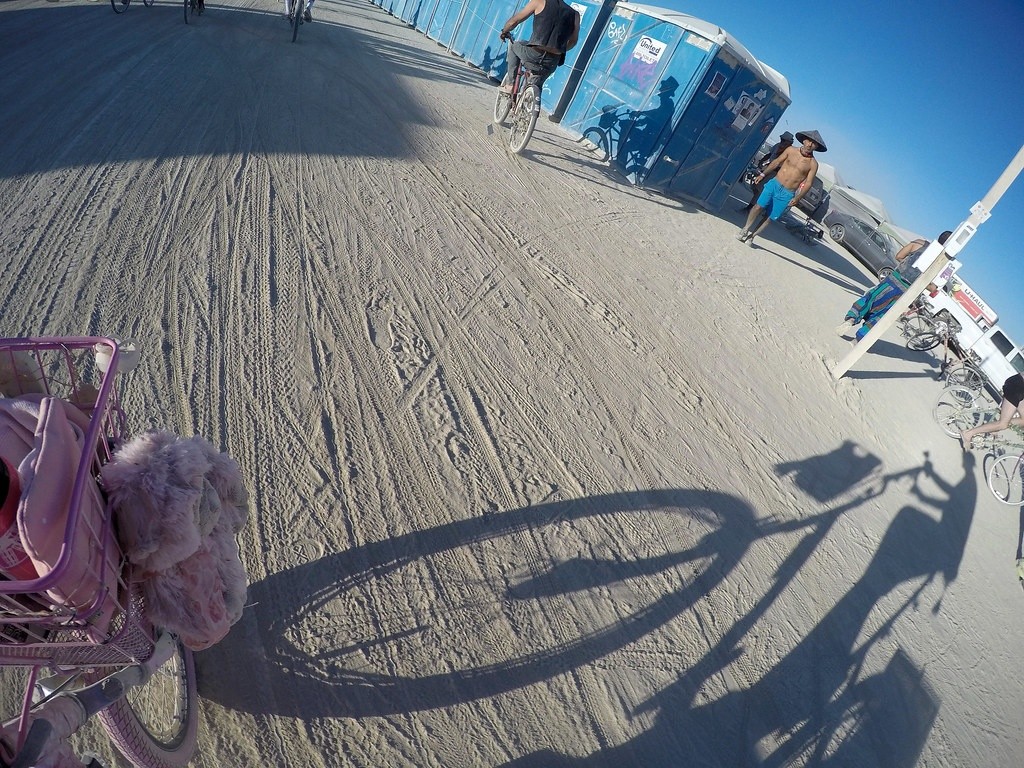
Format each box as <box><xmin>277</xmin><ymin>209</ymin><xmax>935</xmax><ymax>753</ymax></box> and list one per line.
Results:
<box><xmin>531</xmin><ymin>45</ymin><xmax>561</xmax><ymax>59</ymax></box>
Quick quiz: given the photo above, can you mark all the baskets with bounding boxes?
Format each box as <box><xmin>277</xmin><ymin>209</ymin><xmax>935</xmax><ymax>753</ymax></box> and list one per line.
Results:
<box><xmin>0</xmin><ymin>336</ymin><xmax>155</xmax><ymax>664</ymax></box>
<box><xmin>506</xmin><ymin>40</ymin><xmax>526</xmax><ymax>66</ymax></box>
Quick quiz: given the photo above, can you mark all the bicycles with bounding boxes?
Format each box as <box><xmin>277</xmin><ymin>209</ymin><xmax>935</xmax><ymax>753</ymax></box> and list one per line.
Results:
<box><xmin>932</xmin><ymin>385</ymin><xmax>1024</xmax><ymax>508</ymax></box>
<box><xmin>110</xmin><ymin>0</ymin><xmax>154</xmax><ymax>13</ymax></box>
<box><xmin>182</xmin><ymin>0</ymin><xmax>205</xmax><ymax>23</ymax></box>
<box><xmin>0</xmin><ymin>329</ymin><xmax>200</xmax><ymax>768</ymax></box>
<box><xmin>289</xmin><ymin>0</ymin><xmax>309</xmax><ymax>42</ymax></box>
<box><xmin>903</xmin><ymin>315</ymin><xmax>984</xmax><ymax>402</ymax></box>
<box><xmin>493</xmin><ymin>32</ymin><xmax>541</xmax><ymax>156</ymax></box>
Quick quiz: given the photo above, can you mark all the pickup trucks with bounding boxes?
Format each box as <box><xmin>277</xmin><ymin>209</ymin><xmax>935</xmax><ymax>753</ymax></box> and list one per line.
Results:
<box><xmin>921</xmin><ymin>289</ymin><xmax>1024</xmax><ymax>398</ymax></box>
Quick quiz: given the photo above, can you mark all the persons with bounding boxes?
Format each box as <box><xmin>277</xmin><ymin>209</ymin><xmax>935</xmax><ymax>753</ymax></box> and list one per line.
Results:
<box><xmin>744</xmin><ymin>131</ymin><xmax>794</xmax><ymax>210</ymax></box>
<box><xmin>281</xmin><ymin>0</ymin><xmax>314</xmax><ymax>23</ymax></box>
<box><xmin>497</xmin><ymin>0</ymin><xmax>580</xmax><ymax>97</ymax></box>
<box><xmin>836</xmin><ymin>231</ymin><xmax>953</xmax><ymax>344</ymax></box>
<box><xmin>737</xmin><ymin>130</ymin><xmax>826</xmax><ymax>248</ymax></box>
<box><xmin>741</xmin><ymin>103</ymin><xmax>754</xmax><ymax>119</ymax></box>
<box><xmin>960</xmin><ymin>371</ymin><xmax>1024</xmax><ymax>451</ymax></box>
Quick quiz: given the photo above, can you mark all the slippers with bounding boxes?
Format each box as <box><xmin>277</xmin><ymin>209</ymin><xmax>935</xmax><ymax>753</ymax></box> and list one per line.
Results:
<box><xmin>959</xmin><ymin>430</ymin><xmax>971</xmax><ymax>453</ymax></box>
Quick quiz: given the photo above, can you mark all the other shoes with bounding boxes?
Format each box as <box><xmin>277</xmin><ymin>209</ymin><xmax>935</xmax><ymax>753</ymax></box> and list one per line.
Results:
<box><xmin>497</xmin><ymin>84</ymin><xmax>513</xmax><ymax>94</ymax></box>
<box><xmin>834</xmin><ymin>322</ymin><xmax>852</xmax><ymax>337</ymax></box>
<box><xmin>303</xmin><ymin>7</ymin><xmax>312</xmax><ymax>23</ymax></box>
<box><xmin>285</xmin><ymin>13</ymin><xmax>291</xmax><ymax>17</ymax></box>
<box><xmin>735</xmin><ymin>208</ymin><xmax>749</xmax><ymax>216</ymax></box>
<box><xmin>734</xmin><ymin>226</ymin><xmax>749</xmax><ymax>241</ymax></box>
<box><xmin>744</xmin><ymin>233</ymin><xmax>754</xmax><ymax>247</ymax></box>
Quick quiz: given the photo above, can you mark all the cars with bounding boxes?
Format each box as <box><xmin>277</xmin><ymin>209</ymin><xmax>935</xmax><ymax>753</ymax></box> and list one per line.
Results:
<box><xmin>822</xmin><ymin>208</ymin><xmax>900</xmax><ymax>285</ymax></box>
<box><xmin>751</xmin><ymin>141</ymin><xmax>823</xmax><ymax>215</ymax></box>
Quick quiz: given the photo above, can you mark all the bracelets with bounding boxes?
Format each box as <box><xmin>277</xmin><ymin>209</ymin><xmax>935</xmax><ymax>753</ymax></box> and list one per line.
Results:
<box><xmin>760</xmin><ymin>173</ymin><xmax>765</xmax><ymax>178</ymax></box>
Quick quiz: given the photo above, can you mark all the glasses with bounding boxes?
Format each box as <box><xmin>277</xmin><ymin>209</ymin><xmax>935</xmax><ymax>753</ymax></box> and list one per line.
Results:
<box><xmin>781</xmin><ymin>138</ymin><xmax>786</xmax><ymax>142</ymax></box>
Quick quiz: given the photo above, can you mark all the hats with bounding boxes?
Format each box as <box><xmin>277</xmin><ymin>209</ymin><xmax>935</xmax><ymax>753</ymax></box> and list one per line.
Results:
<box><xmin>795</xmin><ymin>130</ymin><xmax>827</xmax><ymax>152</ymax></box>
<box><xmin>780</xmin><ymin>131</ymin><xmax>794</xmax><ymax>141</ymax></box>
